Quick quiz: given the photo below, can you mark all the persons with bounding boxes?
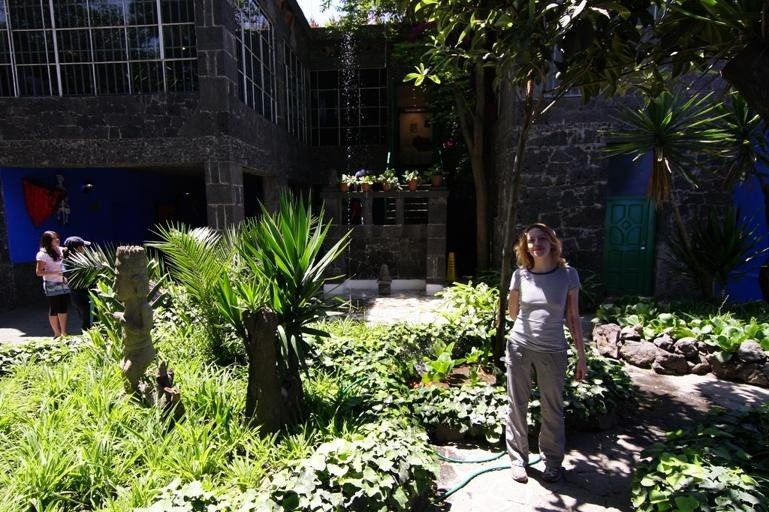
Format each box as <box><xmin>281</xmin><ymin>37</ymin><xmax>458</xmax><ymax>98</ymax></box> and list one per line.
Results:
<box><xmin>36</xmin><ymin>230</ymin><xmax>70</xmax><ymax>340</ymax></box>
<box><xmin>63</xmin><ymin>237</ymin><xmax>100</xmax><ymax>331</ymax></box>
<box><xmin>504</xmin><ymin>221</ymin><xmax>585</xmax><ymax>481</ymax></box>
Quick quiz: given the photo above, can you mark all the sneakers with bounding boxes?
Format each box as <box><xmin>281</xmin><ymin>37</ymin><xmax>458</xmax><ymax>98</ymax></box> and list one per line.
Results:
<box><xmin>544</xmin><ymin>465</ymin><xmax>561</xmax><ymax>482</ymax></box>
<box><xmin>511</xmin><ymin>465</ymin><xmax>528</xmax><ymax>483</ymax></box>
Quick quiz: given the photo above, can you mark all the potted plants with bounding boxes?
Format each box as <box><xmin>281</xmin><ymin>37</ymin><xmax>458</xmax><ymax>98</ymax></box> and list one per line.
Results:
<box><xmin>334</xmin><ymin>162</ymin><xmax>451</xmax><ymax>194</ymax></box>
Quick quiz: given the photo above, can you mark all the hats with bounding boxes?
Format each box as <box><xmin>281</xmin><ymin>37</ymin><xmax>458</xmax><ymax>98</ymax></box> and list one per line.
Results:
<box><xmin>64</xmin><ymin>236</ymin><xmax>91</xmax><ymax>246</ymax></box>
